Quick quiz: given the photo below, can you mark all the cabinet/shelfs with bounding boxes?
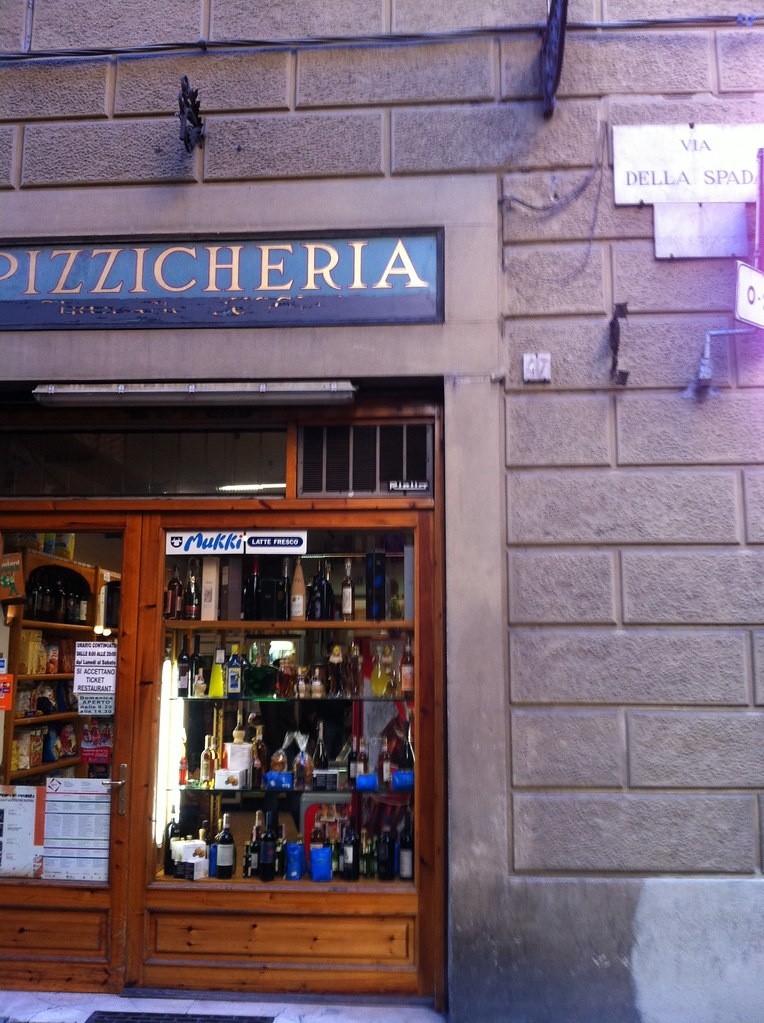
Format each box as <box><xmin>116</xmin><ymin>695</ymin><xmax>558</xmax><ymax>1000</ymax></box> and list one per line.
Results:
<box><xmin>155</xmin><ymin>529</ymin><xmax>417</xmax><ymax>886</ymax></box>
<box><xmin>0</xmin><ymin>548</ymin><xmax>124</xmax><ymax>786</ymax></box>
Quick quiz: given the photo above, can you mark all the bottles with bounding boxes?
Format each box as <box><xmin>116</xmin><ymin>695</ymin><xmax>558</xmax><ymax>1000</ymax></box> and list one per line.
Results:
<box><xmin>4</xmin><ymin>524</ymin><xmax>415</xmax><ymax>883</ymax></box>
